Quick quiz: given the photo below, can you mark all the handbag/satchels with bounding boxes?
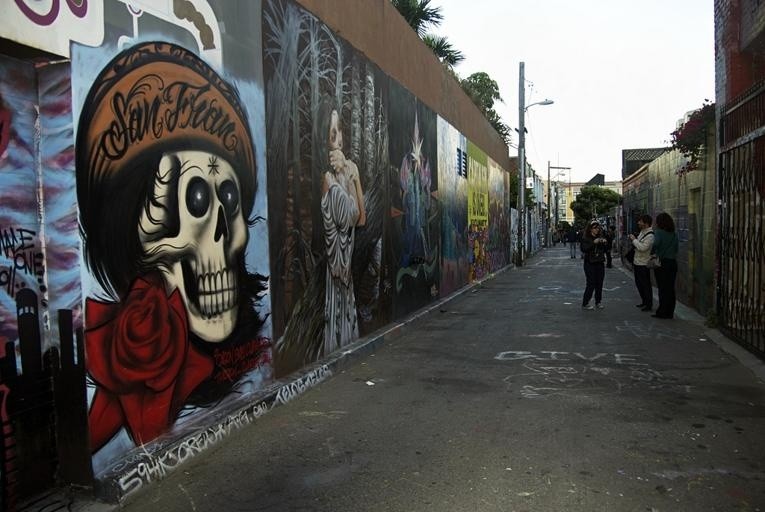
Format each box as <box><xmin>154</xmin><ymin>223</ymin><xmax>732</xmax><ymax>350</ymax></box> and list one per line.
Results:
<box><xmin>589</xmin><ymin>249</ymin><xmax>605</xmax><ymax>264</ymax></box>
<box><xmin>646</xmin><ymin>253</ymin><xmax>661</xmax><ymax>270</ymax></box>
<box><xmin>624</xmin><ymin>248</ymin><xmax>635</xmax><ymax>263</ymax></box>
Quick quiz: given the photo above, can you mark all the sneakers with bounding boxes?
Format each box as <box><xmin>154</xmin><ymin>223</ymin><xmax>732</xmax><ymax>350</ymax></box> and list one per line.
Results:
<box><xmin>582</xmin><ymin>303</ymin><xmax>603</xmax><ymax>310</ymax></box>
<box><xmin>571</xmin><ymin>254</ymin><xmax>584</xmax><ymax>259</ymax></box>
<box><xmin>636</xmin><ymin>302</ymin><xmax>673</xmax><ymax>319</ymax></box>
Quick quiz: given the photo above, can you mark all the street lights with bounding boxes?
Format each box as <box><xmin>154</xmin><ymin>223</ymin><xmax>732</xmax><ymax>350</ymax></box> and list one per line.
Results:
<box><xmin>518</xmin><ymin>60</ymin><xmax>554</xmax><ymax>267</ymax></box>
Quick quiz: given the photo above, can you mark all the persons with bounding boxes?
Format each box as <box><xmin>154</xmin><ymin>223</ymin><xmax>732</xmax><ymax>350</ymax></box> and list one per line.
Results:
<box><xmin>316</xmin><ymin>102</ymin><xmax>367</xmax><ymax>356</ymax></box>
<box><xmin>561</xmin><ymin>225</ymin><xmax>617</xmax><ymax>269</ymax></box>
<box><xmin>580</xmin><ymin>220</ymin><xmax>609</xmax><ymax>310</ymax></box>
<box><xmin>630</xmin><ymin>214</ymin><xmax>656</xmax><ymax>312</ymax></box>
<box><xmin>650</xmin><ymin>212</ymin><xmax>680</xmax><ymax>320</ymax></box>
